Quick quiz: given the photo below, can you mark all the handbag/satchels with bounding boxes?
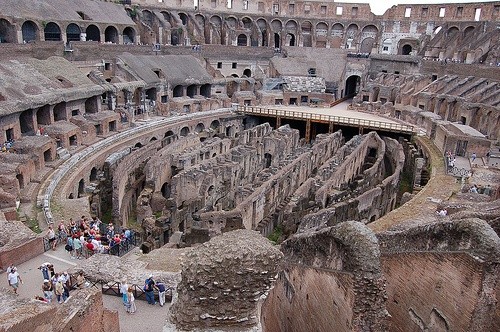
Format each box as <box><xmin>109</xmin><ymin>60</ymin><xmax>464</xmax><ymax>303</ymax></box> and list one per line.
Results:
<box><xmin>65</xmin><ymin>245</ymin><xmax>72</xmax><ymax>251</ymax></box>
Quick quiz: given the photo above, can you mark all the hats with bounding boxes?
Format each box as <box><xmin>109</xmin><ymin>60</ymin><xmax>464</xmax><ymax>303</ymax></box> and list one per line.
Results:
<box><xmin>58</xmin><ymin>277</ymin><xmax>63</xmax><ymax>281</ymax></box>
<box><xmin>44</xmin><ymin>279</ymin><xmax>48</xmax><ymax>282</ymax></box>
<box><xmin>72</xmin><ymin>234</ymin><xmax>79</xmax><ymax>238</ymax></box>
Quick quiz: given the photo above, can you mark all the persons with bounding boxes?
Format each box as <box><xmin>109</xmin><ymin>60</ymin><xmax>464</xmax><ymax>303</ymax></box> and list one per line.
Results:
<box><xmin>126</xmin><ymin>287</ymin><xmax>137</xmax><ymax>314</ymax></box>
<box><xmin>40</xmin><ymin>126</ymin><xmax>44</xmax><ymax>135</ymax></box>
<box><xmin>37</xmin><ymin>262</ymin><xmax>71</xmax><ymax>305</ymax></box>
<box><xmin>7</xmin><ymin>263</ymin><xmax>24</xmax><ymax>295</ymax></box>
<box><xmin>77</xmin><ymin>272</ymin><xmax>85</xmax><ymax>287</ymax></box>
<box><xmin>121</xmin><ymin>280</ymin><xmax>128</xmax><ymax>306</ymax></box>
<box><xmin>144</xmin><ymin>275</ymin><xmax>156</xmax><ymax>305</ymax></box>
<box><xmin>0</xmin><ymin>138</ymin><xmax>15</xmax><ymax>153</ymax></box>
<box><xmin>155</xmin><ymin>279</ymin><xmax>166</xmax><ymax>307</ymax></box>
<box><xmin>48</xmin><ymin>216</ymin><xmax>130</xmax><ymax>259</ymax></box>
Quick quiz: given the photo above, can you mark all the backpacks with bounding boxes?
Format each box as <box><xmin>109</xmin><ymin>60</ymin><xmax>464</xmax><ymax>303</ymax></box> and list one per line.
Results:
<box><xmin>144</xmin><ymin>279</ymin><xmax>151</xmax><ymax>290</ymax></box>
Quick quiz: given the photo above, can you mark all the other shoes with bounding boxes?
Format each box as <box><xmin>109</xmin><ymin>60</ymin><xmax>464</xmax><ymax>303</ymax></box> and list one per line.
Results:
<box><xmin>53</xmin><ymin>248</ymin><xmax>56</xmax><ymax>251</ymax></box>
<box><xmin>160</xmin><ymin>304</ymin><xmax>163</xmax><ymax>307</ymax></box>
